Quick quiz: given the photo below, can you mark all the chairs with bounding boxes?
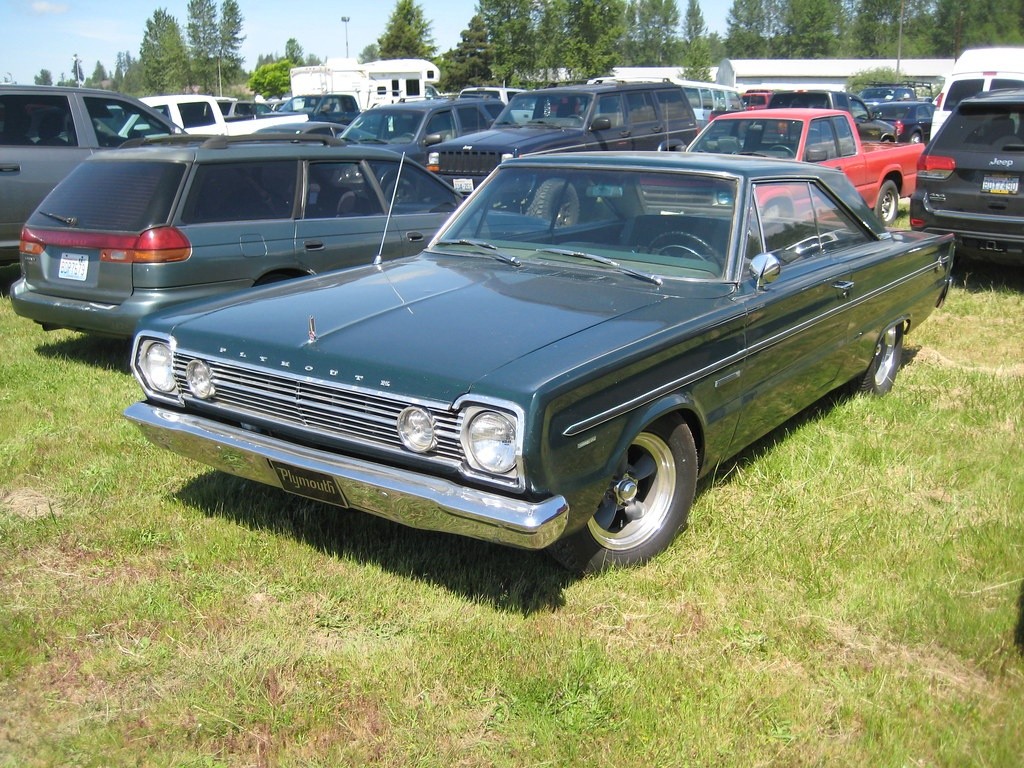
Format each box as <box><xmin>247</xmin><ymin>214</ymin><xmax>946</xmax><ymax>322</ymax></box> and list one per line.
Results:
<box><xmin>556</xmin><ymin>103</ymin><xmax>575</xmax><ymax>120</ymax></box>
<box><xmin>794</xmin><ymin>128</ymin><xmax>819</xmax><ymax>157</ymax></box>
<box><xmin>196</xmin><ymin>168</ymin><xmax>292</xmax><ymax>219</ymax></box>
<box><xmin>741</xmin><ymin>125</ymin><xmax>764</xmax><ymax>151</ymax></box>
<box><xmin>973</xmin><ymin>117</ymin><xmax>1015</xmax><ymax>147</ymax></box>
<box><xmin>621</xmin><ymin>214</ymin><xmax>731</xmax><ymax>270</ymax></box>
<box><xmin>0</xmin><ymin>113</ymin><xmax>70</xmax><ymax>146</ymax></box>
<box><xmin>599</xmin><ymin>99</ymin><xmax>689</xmax><ymax>127</ymax></box>
<box><xmin>317</xmin><ymin>185</ymin><xmax>358</xmax><ymax>216</ymax></box>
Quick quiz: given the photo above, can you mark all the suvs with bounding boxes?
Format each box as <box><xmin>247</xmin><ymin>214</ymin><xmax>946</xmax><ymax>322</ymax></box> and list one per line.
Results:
<box><xmin>909</xmin><ymin>87</ymin><xmax>1024</xmax><ymax>274</ymax></box>
<box><xmin>0</xmin><ymin>81</ymin><xmax>189</xmax><ymax>271</ymax></box>
<box><xmin>10</xmin><ymin>134</ymin><xmax>561</xmax><ymax>340</ymax></box>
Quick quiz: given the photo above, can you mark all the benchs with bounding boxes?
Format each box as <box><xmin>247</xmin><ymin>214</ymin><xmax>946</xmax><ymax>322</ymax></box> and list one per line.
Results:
<box><xmin>748</xmin><ymin>216</ymin><xmax>835</xmax><ymax>262</ymax></box>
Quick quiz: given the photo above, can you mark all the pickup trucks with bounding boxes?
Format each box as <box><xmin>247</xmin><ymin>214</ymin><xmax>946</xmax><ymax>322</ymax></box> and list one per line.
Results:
<box><xmin>97</xmin><ymin>94</ymin><xmax>310</xmax><ymax>138</ymax></box>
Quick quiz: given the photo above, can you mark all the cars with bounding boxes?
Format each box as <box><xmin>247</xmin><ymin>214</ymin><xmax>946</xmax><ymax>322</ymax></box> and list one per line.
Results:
<box><xmin>262</xmin><ymin>75</ymin><xmax>939</xmax><ymax>220</ymax></box>
<box><xmin>118</xmin><ymin>148</ymin><xmax>958</xmax><ymax>573</ymax></box>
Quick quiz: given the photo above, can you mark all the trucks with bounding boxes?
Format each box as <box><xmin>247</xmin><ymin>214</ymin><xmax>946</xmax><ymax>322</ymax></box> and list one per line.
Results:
<box><xmin>930</xmin><ymin>45</ymin><xmax>1024</xmax><ymax>147</ymax></box>
